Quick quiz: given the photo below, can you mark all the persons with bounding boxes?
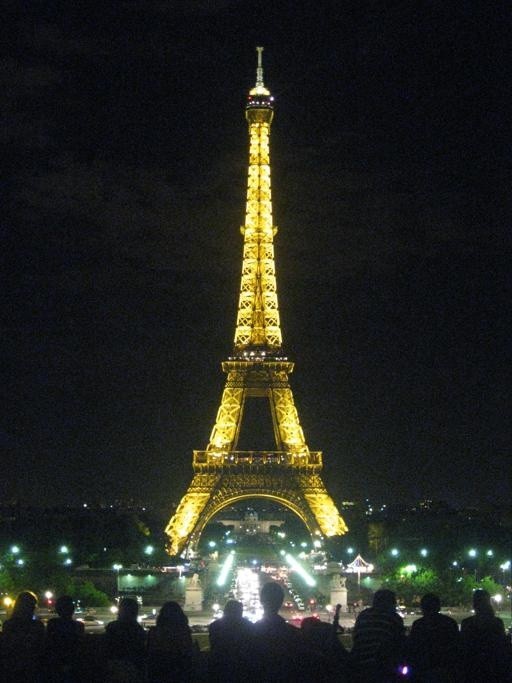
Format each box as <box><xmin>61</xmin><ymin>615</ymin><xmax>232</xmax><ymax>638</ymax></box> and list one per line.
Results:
<box><xmin>250</xmin><ymin>581</ymin><xmax>301</xmax><ymax>683</ymax></box>
<box><xmin>45</xmin><ymin>594</ymin><xmax>86</xmax><ymax>682</ymax></box>
<box><xmin>295</xmin><ymin>616</ymin><xmax>350</xmax><ymax>683</ymax></box>
<box><xmin>101</xmin><ymin>597</ymin><xmax>148</xmax><ymax>681</ymax></box>
<box><xmin>349</xmin><ymin>588</ymin><xmax>406</xmax><ymax>681</ymax></box>
<box><xmin>0</xmin><ymin>589</ymin><xmax>46</xmax><ymax>682</ymax></box>
<box><xmin>332</xmin><ymin>604</ymin><xmax>345</xmax><ymax>632</ymax></box>
<box><xmin>406</xmin><ymin>592</ymin><xmax>460</xmax><ymax>681</ymax></box>
<box><xmin>145</xmin><ymin>600</ymin><xmax>198</xmax><ymax>682</ymax></box>
<box><xmin>207</xmin><ymin>598</ymin><xmax>255</xmax><ymax>681</ymax></box>
<box><xmin>459</xmin><ymin>587</ymin><xmax>510</xmax><ymax>683</ymax></box>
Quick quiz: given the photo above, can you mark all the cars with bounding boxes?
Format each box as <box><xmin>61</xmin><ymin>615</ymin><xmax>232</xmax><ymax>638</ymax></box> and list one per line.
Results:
<box><xmin>74</xmin><ymin>608</ymin><xmax>103</xmax><ymax>626</ymax></box>
<box><xmin>137</xmin><ymin>613</ymin><xmax>157</xmax><ymax>628</ymax></box>
<box><xmin>285</xmin><ymin>583</ymin><xmax>305</xmax><ymax>612</ymax></box>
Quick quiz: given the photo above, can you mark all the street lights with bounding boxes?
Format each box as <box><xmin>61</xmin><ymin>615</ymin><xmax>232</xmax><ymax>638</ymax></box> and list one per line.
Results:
<box><xmin>113</xmin><ymin>564</ymin><xmax>122</xmax><ymax>589</ymax></box>
